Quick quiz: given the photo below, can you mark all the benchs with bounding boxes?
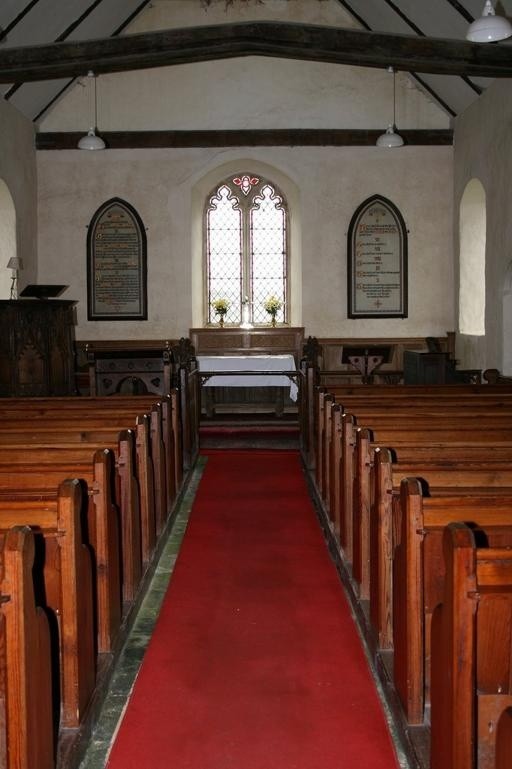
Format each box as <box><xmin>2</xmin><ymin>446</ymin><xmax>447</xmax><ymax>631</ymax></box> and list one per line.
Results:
<box><xmin>303</xmin><ymin>385</ymin><xmax>512</xmax><ymax>768</ymax></box>
<box><xmin>0</xmin><ymin>399</ymin><xmax>188</xmax><ymax>769</ymax></box>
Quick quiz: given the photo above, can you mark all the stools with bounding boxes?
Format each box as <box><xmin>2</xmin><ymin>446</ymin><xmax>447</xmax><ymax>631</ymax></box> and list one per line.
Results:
<box><xmin>456</xmin><ymin>369</ymin><xmax>482</xmax><ymax>384</ymax></box>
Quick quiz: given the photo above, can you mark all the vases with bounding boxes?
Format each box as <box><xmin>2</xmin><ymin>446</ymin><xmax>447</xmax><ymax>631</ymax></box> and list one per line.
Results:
<box><xmin>270</xmin><ymin>314</ymin><xmax>277</xmax><ymax>328</ymax></box>
<box><xmin>219</xmin><ymin>314</ymin><xmax>225</xmax><ymax>327</ymax></box>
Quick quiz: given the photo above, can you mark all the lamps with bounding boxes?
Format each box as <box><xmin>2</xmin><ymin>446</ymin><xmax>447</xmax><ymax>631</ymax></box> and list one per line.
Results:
<box><xmin>376</xmin><ymin>68</ymin><xmax>405</xmax><ymax>147</ymax></box>
<box><xmin>465</xmin><ymin>0</ymin><xmax>512</xmax><ymax>43</ymax></box>
<box><xmin>77</xmin><ymin>69</ymin><xmax>105</xmax><ymax>150</ymax></box>
<box><xmin>7</xmin><ymin>256</ymin><xmax>24</xmax><ymax>299</ymax></box>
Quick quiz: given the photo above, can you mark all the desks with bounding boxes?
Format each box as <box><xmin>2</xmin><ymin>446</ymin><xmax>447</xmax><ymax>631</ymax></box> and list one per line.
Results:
<box><xmin>197</xmin><ymin>355</ymin><xmax>297</xmax><ymax>415</ymax></box>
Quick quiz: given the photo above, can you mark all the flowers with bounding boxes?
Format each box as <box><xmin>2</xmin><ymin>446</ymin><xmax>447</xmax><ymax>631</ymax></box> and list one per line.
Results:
<box><xmin>210</xmin><ymin>297</ymin><xmax>230</xmax><ymax>317</ymax></box>
<box><xmin>262</xmin><ymin>294</ymin><xmax>286</xmax><ymax>316</ymax></box>
